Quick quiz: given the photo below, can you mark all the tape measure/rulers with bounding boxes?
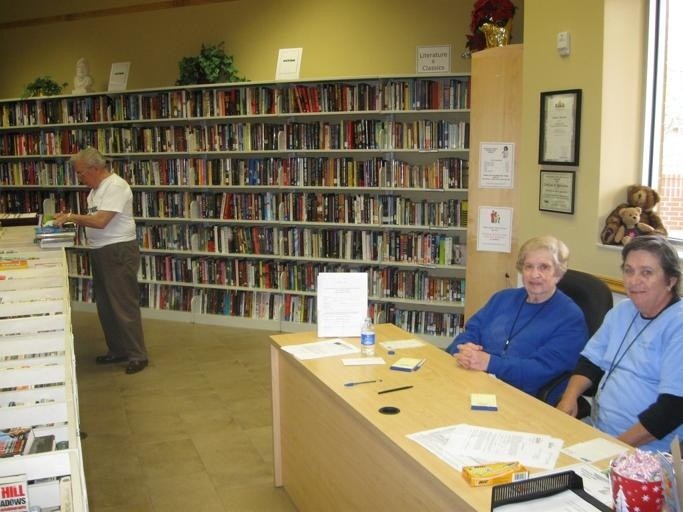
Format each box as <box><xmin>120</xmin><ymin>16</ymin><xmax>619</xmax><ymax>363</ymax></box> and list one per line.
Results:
<box><xmin>655</xmin><ymin>434</ymin><xmax>683</xmax><ymax>512</ymax></box>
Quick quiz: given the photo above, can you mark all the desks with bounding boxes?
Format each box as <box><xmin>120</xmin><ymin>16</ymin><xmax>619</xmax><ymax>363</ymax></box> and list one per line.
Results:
<box><xmin>266</xmin><ymin>321</ymin><xmax>682</xmax><ymax>511</ymax></box>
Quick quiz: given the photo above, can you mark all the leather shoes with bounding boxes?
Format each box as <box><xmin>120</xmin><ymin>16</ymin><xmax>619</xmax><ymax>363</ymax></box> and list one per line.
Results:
<box><xmin>126</xmin><ymin>358</ymin><xmax>148</xmax><ymax>374</ymax></box>
<box><xmin>96</xmin><ymin>351</ymin><xmax>126</xmax><ymax>364</ymax></box>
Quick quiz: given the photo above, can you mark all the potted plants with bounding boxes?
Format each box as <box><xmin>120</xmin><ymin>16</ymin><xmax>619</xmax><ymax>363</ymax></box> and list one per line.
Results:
<box><xmin>19</xmin><ymin>75</ymin><xmax>67</xmax><ymax>100</ymax></box>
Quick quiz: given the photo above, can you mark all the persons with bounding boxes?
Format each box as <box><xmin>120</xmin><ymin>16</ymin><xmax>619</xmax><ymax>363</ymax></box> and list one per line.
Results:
<box><xmin>446</xmin><ymin>234</ymin><xmax>591</xmax><ymax>396</ymax></box>
<box><xmin>53</xmin><ymin>147</ymin><xmax>150</xmax><ymax>374</ymax></box>
<box><xmin>555</xmin><ymin>235</ymin><xmax>683</xmax><ymax>461</ymax></box>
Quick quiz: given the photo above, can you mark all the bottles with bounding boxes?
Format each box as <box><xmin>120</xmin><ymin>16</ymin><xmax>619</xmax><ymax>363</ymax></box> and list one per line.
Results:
<box><xmin>362</xmin><ymin>315</ymin><xmax>376</xmax><ymax>356</ymax></box>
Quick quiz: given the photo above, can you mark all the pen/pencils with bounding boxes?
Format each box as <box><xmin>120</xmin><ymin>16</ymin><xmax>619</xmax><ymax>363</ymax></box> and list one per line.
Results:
<box><xmin>378</xmin><ymin>386</ymin><xmax>413</xmax><ymax>394</ymax></box>
<box><xmin>344</xmin><ymin>380</ymin><xmax>382</xmax><ymax>386</ymax></box>
<box><xmin>414</xmin><ymin>358</ymin><xmax>426</xmax><ymax>371</ymax></box>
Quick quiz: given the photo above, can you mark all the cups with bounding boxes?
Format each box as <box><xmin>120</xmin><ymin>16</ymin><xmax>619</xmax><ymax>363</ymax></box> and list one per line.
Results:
<box><xmin>612</xmin><ymin>467</ymin><xmax>675</xmax><ymax>512</ymax></box>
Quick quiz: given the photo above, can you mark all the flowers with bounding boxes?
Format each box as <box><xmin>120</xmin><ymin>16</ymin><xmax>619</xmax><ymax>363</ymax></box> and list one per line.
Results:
<box><xmin>462</xmin><ymin>0</ymin><xmax>518</xmax><ymax>54</ymax></box>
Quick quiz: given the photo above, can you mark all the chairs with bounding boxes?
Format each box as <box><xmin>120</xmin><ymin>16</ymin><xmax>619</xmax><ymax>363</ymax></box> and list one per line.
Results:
<box><xmin>534</xmin><ymin>269</ymin><xmax>613</xmax><ymax>419</ymax></box>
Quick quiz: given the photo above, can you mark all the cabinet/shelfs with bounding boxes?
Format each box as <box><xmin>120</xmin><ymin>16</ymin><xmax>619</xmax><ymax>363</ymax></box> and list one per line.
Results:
<box><xmin>0</xmin><ymin>74</ymin><xmax>472</xmax><ymax>350</ymax></box>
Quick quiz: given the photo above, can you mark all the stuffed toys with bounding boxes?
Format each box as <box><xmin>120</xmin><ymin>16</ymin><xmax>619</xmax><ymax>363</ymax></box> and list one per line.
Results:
<box><xmin>615</xmin><ymin>206</ymin><xmax>655</xmax><ymax>246</ymax></box>
<box><xmin>601</xmin><ymin>185</ymin><xmax>669</xmax><ymax>245</ymax></box>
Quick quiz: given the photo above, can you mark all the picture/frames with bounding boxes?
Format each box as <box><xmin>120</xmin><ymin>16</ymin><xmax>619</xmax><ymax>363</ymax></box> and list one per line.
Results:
<box><xmin>537</xmin><ymin>88</ymin><xmax>582</xmax><ymax>168</ymax></box>
<box><xmin>538</xmin><ymin>170</ymin><xmax>575</xmax><ymax>215</ymax></box>
